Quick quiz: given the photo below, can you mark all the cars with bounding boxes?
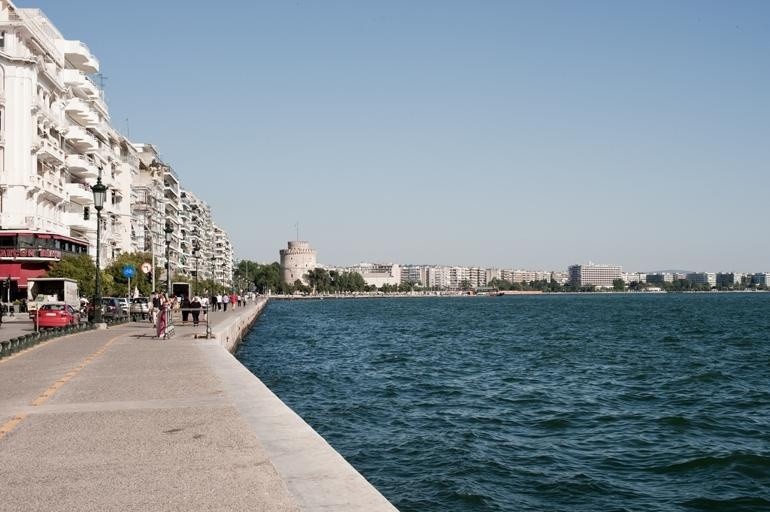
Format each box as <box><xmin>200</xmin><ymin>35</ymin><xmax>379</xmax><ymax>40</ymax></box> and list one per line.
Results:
<box><xmin>88</xmin><ymin>296</ymin><xmax>151</xmax><ymax>320</ymax></box>
<box><xmin>33</xmin><ymin>303</ymin><xmax>81</xmax><ymax>331</ymax></box>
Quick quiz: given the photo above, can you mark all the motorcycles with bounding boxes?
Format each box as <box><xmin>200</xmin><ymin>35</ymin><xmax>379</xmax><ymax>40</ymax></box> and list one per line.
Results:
<box><xmin>80</xmin><ymin>301</ymin><xmax>88</xmax><ymax>318</ymax></box>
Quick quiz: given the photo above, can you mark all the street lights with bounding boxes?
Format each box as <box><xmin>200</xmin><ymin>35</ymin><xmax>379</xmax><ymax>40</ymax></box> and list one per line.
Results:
<box><xmin>89</xmin><ymin>176</ymin><xmax>108</xmax><ymax>319</ymax></box>
<box><xmin>193</xmin><ymin>242</ymin><xmax>255</xmax><ymax>297</ymax></box>
<box><xmin>163</xmin><ymin>224</ymin><xmax>174</xmax><ymax>298</ymax></box>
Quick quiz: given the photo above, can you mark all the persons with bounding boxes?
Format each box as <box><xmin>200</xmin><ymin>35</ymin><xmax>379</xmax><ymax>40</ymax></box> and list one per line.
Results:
<box><xmin>81</xmin><ymin>294</ymin><xmax>89</xmax><ymax>305</ymax></box>
<box><xmin>148</xmin><ymin>290</ymin><xmax>260</xmax><ymax>340</ymax></box>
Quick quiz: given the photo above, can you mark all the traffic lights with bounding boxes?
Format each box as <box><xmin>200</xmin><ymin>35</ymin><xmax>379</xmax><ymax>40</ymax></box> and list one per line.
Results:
<box><xmin>2</xmin><ymin>279</ymin><xmax>7</xmax><ymax>289</ymax></box>
<box><xmin>83</xmin><ymin>207</ymin><xmax>89</xmax><ymax>220</ymax></box>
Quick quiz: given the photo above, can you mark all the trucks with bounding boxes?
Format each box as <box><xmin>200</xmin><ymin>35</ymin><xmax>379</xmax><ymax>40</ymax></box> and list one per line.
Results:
<box><xmin>171</xmin><ymin>282</ymin><xmax>191</xmax><ymax>307</ymax></box>
<box><xmin>26</xmin><ymin>277</ymin><xmax>80</xmax><ymax>319</ymax></box>
